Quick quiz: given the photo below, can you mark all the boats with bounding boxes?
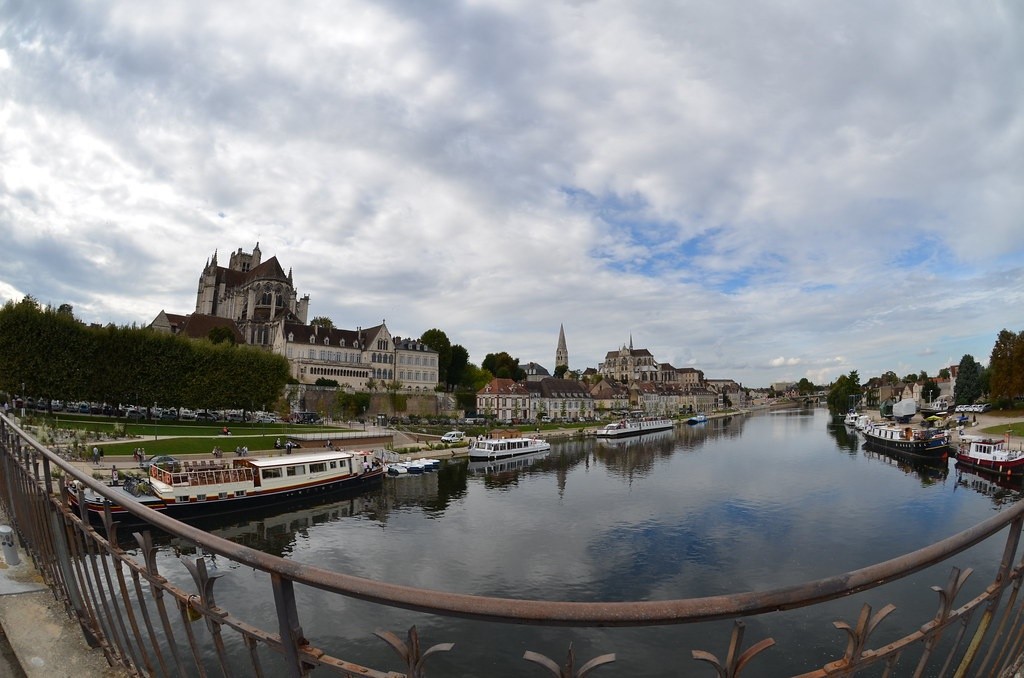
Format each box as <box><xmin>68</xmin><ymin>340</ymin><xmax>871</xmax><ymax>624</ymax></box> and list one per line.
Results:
<box><xmin>596</xmin><ymin>411</ymin><xmax>674</xmax><ymax>439</ymax></box>
<box><xmin>955</xmin><ymin>424</ymin><xmax>1024</xmax><ymax>475</ymax></box>
<box><xmin>862</xmin><ymin>416</ymin><xmax>952</xmax><ymax>460</ymax></box>
<box><xmin>955</xmin><ymin>462</ymin><xmax>1024</xmax><ymax>508</ymax></box>
<box><xmin>466</xmin><ymin>437</ymin><xmax>551</xmax><ymax>460</ymax></box>
<box><xmin>686</xmin><ymin>413</ymin><xmax>707</xmax><ymax>424</ymax></box>
<box><xmin>879</xmin><ymin>398</ymin><xmax>969</xmax><ymax>428</ymax></box>
<box><xmin>597</xmin><ymin>430</ymin><xmax>674</xmax><ymax>452</ymax></box>
<box><xmin>862</xmin><ymin>439</ymin><xmax>949</xmax><ymax>488</ymax></box>
<box><xmin>466</xmin><ymin>451</ymin><xmax>551</xmax><ymax>478</ymax></box>
<box><xmin>389</xmin><ymin>459</ymin><xmax>440</xmax><ymax>475</ymax></box>
<box><xmin>844</xmin><ymin>412</ymin><xmax>872</xmax><ymax>430</ymax></box>
<box><xmin>64</xmin><ymin>451</ymin><xmax>389</xmax><ymax>526</ymax></box>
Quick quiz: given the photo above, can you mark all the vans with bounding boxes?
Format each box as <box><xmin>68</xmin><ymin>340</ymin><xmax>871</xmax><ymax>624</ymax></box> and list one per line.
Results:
<box><xmin>440</xmin><ymin>431</ymin><xmax>465</xmax><ymax>443</ymax></box>
<box><xmin>931</xmin><ymin>402</ymin><xmax>948</xmax><ymax>411</ymax></box>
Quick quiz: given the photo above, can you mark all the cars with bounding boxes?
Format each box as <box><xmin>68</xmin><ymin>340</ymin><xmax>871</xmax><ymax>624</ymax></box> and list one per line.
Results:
<box><xmin>12</xmin><ymin>397</ymin><xmax>323</xmax><ymax>426</ymax></box>
<box><xmin>140</xmin><ymin>454</ymin><xmax>179</xmax><ymax>471</ymax></box>
<box><xmin>955</xmin><ymin>404</ymin><xmax>993</xmax><ymax>413</ymax></box>
<box><xmin>388</xmin><ymin>415</ymin><xmax>602</xmax><ymax>426</ymax></box>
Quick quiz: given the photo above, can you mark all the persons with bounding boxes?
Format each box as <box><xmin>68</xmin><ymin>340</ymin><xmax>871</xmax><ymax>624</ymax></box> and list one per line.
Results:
<box><xmin>321</xmin><ymin>438</ymin><xmax>341</xmax><ymax>452</ymax></box>
<box><xmin>112</xmin><ymin>464</ymin><xmax>119</xmax><ymax>486</ymax></box>
<box><xmin>476</xmin><ymin>433</ymin><xmax>492</xmax><ymax>441</ymax></box>
<box><xmin>134</xmin><ymin>447</ymin><xmax>146</xmax><ymax>462</ymax></box>
<box><xmin>213</xmin><ymin>445</ymin><xmax>223</xmax><ymax>458</ymax></box>
<box><xmin>93</xmin><ymin>445</ymin><xmax>104</xmax><ymax>466</ymax></box>
<box><xmin>274</xmin><ymin>437</ymin><xmax>301</xmax><ymax>455</ymax></box>
<box><xmin>236</xmin><ymin>445</ymin><xmax>248</xmax><ymax>456</ymax></box>
<box><xmin>224</xmin><ymin>425</ymin><xmax>232</xmax><ymax>436</ymax></box>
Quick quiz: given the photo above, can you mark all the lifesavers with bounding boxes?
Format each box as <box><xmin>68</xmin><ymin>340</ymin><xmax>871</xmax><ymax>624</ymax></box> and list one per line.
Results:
<box><xmin>166</xmin><ymin>475</ymin><xmax>171</xmax><ymax>483</ymax></box>
<box><xmin>152</xmin><ymin>466</ymin><xmax>157</xmax><ymax>476</ymax></box>
